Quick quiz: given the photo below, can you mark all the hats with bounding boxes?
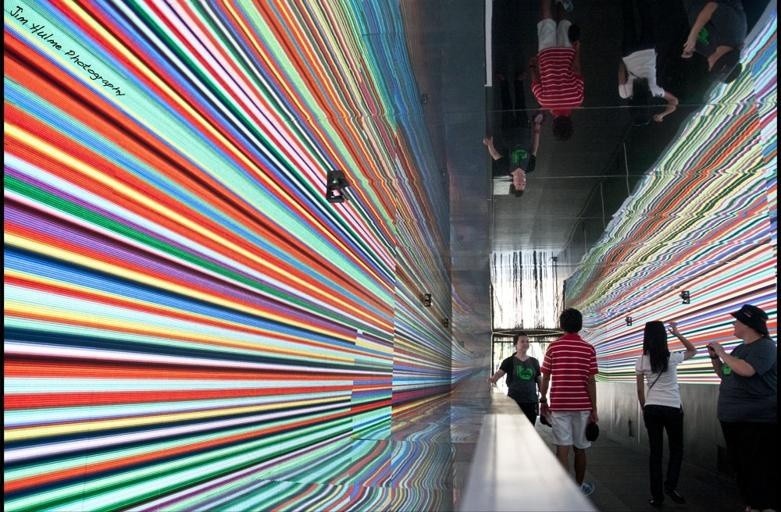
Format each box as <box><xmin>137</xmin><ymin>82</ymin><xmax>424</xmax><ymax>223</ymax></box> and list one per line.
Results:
<box><xmin>729</xmin><ymin>304</ymin><xmax>769</xmax><ymax>334</ymax></box>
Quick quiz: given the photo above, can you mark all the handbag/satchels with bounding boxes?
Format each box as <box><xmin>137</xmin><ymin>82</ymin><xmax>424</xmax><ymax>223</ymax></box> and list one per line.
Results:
<box><xmin>585</xmin><ymin>423</ymin><xmax>599</xmax><ymax>441</ymax></box>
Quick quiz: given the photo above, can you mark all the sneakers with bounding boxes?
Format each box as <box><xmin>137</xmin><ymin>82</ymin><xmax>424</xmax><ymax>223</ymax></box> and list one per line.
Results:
<box><xmin>581</xmin><ymin>482</ymin><xmax>595</xmax><ymax>497</ymax></box>
<box><xmin>649</xmin><ymin>486</ymin><xmax>684</xmax><ymax>505</ymax></box>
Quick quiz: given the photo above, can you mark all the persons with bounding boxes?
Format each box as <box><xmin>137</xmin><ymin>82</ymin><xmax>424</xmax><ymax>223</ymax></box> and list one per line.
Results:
<box><xmin>635</xmin><ymin>320</ymin><xmax>698</xmax><ymax>508</ymax></box>
<box><xmin>481</xmin><ymin>59</ymin><xmax>544</xmax><ymax>204</ymax></box>
<box><xmin>680</xmin><ymin>0</ymin><xmax>748</xmax><ymax>86</ymax></box>
<box><xmin>707</xmin><ymin>305</ymin><xmax>781</xmax><ymax>512</ymax></box>
<box><xmin>540</xmin><ymin>307</ymin><xmax>599</xmax><ymax>497</ymax></box>
<box><xmin>528</xmin><ymin>0</ymin><xmax>586</xmax><ymax>140</ymax></box>
<box><xmin>612</xmin><ymin>0</ymin><xmax>680</xmax><ymax>128</ymax></box>
<box><xmin>488</xmin><ymin>333</ymin><xmax>544</xmax><ymax>427</ymax></box>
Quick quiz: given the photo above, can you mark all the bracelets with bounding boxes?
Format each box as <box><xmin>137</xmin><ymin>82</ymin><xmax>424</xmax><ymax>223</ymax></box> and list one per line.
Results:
<box><xmin>540</xmin><ymin>397</ymin><xmax>547</xmax><ymax>403</ymax></box>
<box><xmin>709</xmin><ymin>354</ymin><xmax>720</xmax><ymax>361</ymax></box>
